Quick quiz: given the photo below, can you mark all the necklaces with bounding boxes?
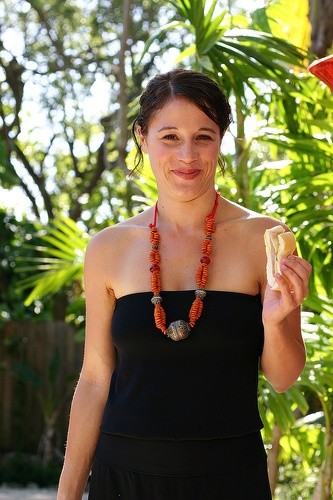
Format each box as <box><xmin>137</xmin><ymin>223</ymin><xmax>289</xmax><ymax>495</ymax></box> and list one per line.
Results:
<box><xmin>148</xmin><ymin>192</ymin><xmax>218</xmax><ymax>341</ymax></box>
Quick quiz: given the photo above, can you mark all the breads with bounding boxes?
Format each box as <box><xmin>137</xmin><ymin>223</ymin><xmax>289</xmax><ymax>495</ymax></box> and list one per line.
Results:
<box><xmin>263</xmin><ymin>225</ymin><xmax>296</xmax><ymax>292</ymax></box>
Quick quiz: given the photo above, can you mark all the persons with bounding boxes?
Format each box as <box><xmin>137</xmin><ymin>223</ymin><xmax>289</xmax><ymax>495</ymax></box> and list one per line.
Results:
<box><xmin>56</xmin><ymin>70</ymin><xmax>313</xmax><ymax>500</ymax></box>
